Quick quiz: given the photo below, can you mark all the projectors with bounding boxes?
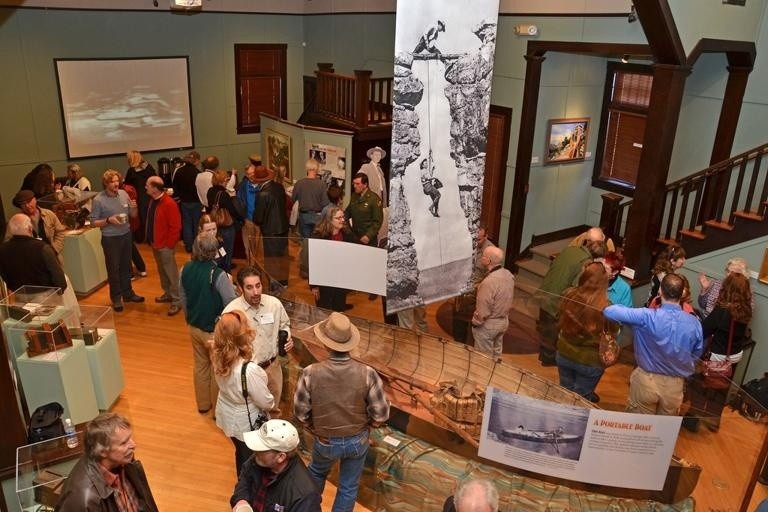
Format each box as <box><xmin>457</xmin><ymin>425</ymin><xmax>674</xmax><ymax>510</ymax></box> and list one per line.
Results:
<box><xmin>171</xmin><ymin>0</ymin><xmax>202</xmax><ymax>15</ymax></box>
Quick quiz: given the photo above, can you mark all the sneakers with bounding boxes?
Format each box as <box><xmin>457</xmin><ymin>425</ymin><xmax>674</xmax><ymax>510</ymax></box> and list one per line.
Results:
<box><xmin>112</xmin><ymin>271</ymin><xmax>182</xmax><ymax>315</ymax></box>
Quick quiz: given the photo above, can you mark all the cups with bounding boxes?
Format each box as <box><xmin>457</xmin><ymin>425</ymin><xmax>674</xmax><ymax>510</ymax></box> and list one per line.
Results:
<box><xmin>118</xmin><ymin>213</ymin><xmax>128</xmax><ymax>224</ymax></box>
<box><xmin>166</xmin><ymin>188</ymin><xmax>173</xmax><ymax>195</ymax></box>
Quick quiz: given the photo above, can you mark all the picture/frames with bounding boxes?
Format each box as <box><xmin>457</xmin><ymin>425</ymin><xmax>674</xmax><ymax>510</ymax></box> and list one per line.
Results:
<box><xmin>542</xmin><ymin>117</ymin><xmax>591</xmax><ymax>165</ymax></box>
<box><xmin>265</xmin><ymin>127</ymin><xmax>292</xmax><ymax>185</ymax></box>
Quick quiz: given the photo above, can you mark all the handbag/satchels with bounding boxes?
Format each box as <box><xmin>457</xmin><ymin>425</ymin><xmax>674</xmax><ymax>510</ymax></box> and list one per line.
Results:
<box><xmin>30</xmin><ymin>402</ymin><xmax>63</xmax><ymax>450</ymax></box>
<box><xmin>211</xmin><ymin>205</ymin><xmax>234</xmax><ymax>226</ymax></box>
<box><xmin>703</xmin><ymin>359</ymin><xmax>733</xmax><ymax>388</ymax></box>
<box><xmin>600</xmin><ymin>332</ymin><xmax>621</xmax><ymax>366</ymax></box>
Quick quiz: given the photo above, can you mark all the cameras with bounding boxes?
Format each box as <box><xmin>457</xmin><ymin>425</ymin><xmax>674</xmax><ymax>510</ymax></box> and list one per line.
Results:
<box><xmin>252</xmin><ymin>415</ymin><xmax>268</xmax><ymax>430</ymax></box>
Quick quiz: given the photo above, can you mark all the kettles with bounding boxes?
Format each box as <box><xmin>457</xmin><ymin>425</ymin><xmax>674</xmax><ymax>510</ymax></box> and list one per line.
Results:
<box><xmin>157</xmin><ymin>157</ymin><xmax>170</xmax><ymax>178</ymax></box>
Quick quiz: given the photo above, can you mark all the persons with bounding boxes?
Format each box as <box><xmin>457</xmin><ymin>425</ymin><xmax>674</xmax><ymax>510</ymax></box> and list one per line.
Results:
<box><xmin>441</xmin><ymin>479</ymin><xmax>502</xmax><ymax>512</ymax></box>
<box><xmin>209</xmin><ymin>310</ymin><xmax>280</xmax><ymax>482</ymax></box>
<box><xmin>230</xmin><ymin>419</ymin><xmax>321</xmax><ymax>512</ymax></box>
<box><xmin>411</xmin><ymin>20</ymin><xmax>445</xmax><ymax>56</ymax></box>
<box><xmin>54</xmin><ymin>413</ymin><xmax>160</xmax><ymax>512</ymax></box>
<box><xmin>420</xmin><ymin>154</ymin><xmax>442</xmax><ymax>218</ymax></box>
<box><xmin>1</xmin><ymin>145</ymin><xmax>386</xmax><ymax>317</ymax></box>
<box><xmin>292</xmin><ymin>312</ymin><xmax>389</xmax><ymax>511</ymax></box>
<box><xmin>178</xmin><ymin>236</ymin><xmax>237</xmax><ymax>415</ymax></box>
<box><xmin>471</xmin><ymin>223</ymin><xmax>753</xmax><ymax>443</ymax></box>
<box><xmin>219</xmin><ymin>266</ymin><xmax>292</xmax><ymax>415</ymax></box>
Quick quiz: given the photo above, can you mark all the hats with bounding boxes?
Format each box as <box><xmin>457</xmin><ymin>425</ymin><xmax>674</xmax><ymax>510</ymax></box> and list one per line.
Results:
<box><xmin>419</xmin><ymin>158</ymin><xmax>427</xmax><ymax>168</ymax></box>
<box><xmin>314</xmin><ymin>312</ymin><xmax>360</xmax><ymax>353</ymax></box>
<box><xmin>16</xmin><ymin>190</ymin><xmax>34</xmax><ymax>203</ymax></box>
<box><xmin>243</xmin><ymin>420</ymin><xmax>300</xmax><ymax>453</ymax></box>
<box><xmin>252</xmin><ymin>165</ymin><xmax>275</xmax><ymax>182</ymax></box>
<box><xmin>366</xmin><ymin>147</ymin><xmax>386</xmax><ymax>161</ymax></box>
<box><xmin>438</xmin><ymin>19</ymin><xmax>447</xmax><ymax>31</ymax></box>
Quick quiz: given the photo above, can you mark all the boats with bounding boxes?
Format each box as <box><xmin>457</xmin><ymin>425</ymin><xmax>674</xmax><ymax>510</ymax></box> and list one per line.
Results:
<box><xmin>260</xmin><ymin>292</ymin><xmax>706</xmax><ymax>505</ymax></box>
<box><xmin>92</xmin><ymin>91</ymin><xmax>184</xmax><ymax>132</ymax></box>
<box><xmin>500</xmin><ymin>427</ymin><xmax>581</xmax><ymax>445</ymax></box>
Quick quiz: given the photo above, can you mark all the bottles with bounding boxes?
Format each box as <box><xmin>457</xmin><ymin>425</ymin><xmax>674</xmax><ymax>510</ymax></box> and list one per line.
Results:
<box><xmin>63</xmin><ymin>418</ymin><xmax>78</xmax><ymax>450</ymax></box>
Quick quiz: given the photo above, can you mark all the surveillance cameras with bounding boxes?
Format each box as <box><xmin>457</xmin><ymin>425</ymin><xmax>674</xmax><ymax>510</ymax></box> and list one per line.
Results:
<box><xmin>513</xmin><ymin>23</ymin><xmax>539</xmax><ymax>37</ymax></box>
